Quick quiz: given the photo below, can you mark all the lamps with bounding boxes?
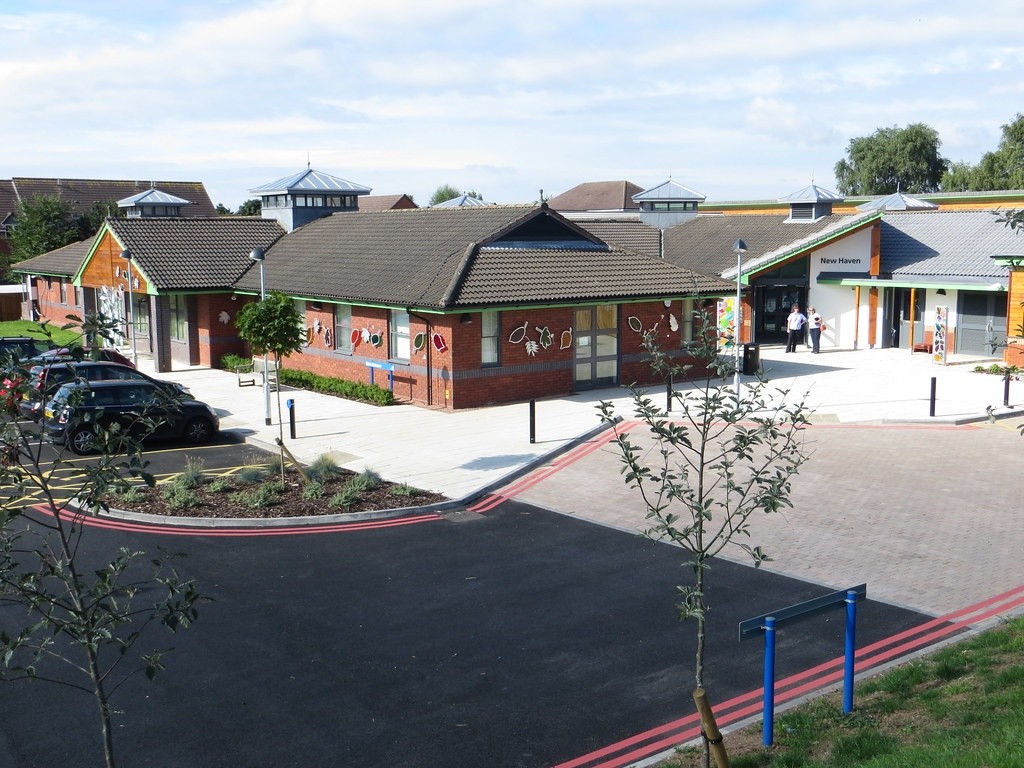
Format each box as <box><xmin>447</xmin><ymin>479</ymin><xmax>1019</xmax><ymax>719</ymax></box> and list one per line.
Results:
<box><xmin>461</xmin><ymin>313</ymin><xmax>473</xmax><ymax>324</ymax></box>
<box><xmin>705</xmin><ymin>299</ymin><xmax>714</xmax><ymax>308</ymax></box>
<box><xmin>311</xmin><ymin>302</ymin><xmax>322</xmax><ymax>310</ymax></box>
<box><xmin>936</xmin><ymin>289</ymin><xmax>946</xmax><ymax>296</ymax></box>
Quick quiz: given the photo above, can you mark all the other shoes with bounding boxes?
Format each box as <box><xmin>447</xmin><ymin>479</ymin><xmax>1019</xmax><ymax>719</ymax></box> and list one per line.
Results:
<box><xmin>814</xmin><ymin>352</ymin><xmax>819</xmax><ymax>354</ymax></box>
<box><xmin>785</xmin><ymin>351</ymin><xmax>789</xmax><ymax>353</ymax></box>
<box><xmin>811</xmin><ymin>351</ymin><xmax>814</xmax><ymax>353</ymax></box>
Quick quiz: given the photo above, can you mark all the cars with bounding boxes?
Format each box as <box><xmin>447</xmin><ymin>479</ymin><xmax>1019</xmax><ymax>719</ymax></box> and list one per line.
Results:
<box><xmin>6</xmin><ymin>334</ymin><xmax>220</xmax><ymax>453</ymax></box>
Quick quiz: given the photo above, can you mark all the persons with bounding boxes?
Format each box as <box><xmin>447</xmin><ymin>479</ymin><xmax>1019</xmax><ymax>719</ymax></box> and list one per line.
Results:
<box><xmin>807</xmin><ymin>307</ymin><xmax>822</xmax><ymax>354</ymax></box>
<box><xmin>785</xmin><ymin>306</ymin><xmax>807</xmax><ymax>353</ymax></box>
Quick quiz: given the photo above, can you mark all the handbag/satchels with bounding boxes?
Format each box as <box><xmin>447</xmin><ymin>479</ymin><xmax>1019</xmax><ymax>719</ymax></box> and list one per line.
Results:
<box><xmin>820</xmin><ymin>324</ymin><xmax>826</xmax><ymax>331</ymax></box>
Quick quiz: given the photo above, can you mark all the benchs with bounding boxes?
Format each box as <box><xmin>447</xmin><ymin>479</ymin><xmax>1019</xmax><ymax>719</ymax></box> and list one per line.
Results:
<box><xmin>914</xmin><ymin>343</ymin><xmax>932</xmax><ymax>353</ymax></box>
<box><xmin>234</xmin><ymin>358</ymin><xmax>282</xmax><ymax>392</ymax></box>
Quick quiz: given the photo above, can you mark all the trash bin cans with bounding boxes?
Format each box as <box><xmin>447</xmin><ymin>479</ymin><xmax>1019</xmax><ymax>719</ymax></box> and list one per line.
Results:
<box><xmin>741</xmin><ymin>343</ymin><xmax>759</xmax><ymax>375</ymax></box>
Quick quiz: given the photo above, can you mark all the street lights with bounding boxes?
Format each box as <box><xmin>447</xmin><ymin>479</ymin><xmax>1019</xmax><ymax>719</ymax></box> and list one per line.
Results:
<box><xmin>249</xmin><ymin>248</ymin><xmax>272</xmax><ymax>426</ymax></box>
<box><xmin>120</xmin><ymin>250</ymin><xmax>141</xmax><ymax>377</ymax></box>
<box><xmin>730</xmin><ymin>239</ymin><xmax>748</xmax><ymax>407</ymax></box>
